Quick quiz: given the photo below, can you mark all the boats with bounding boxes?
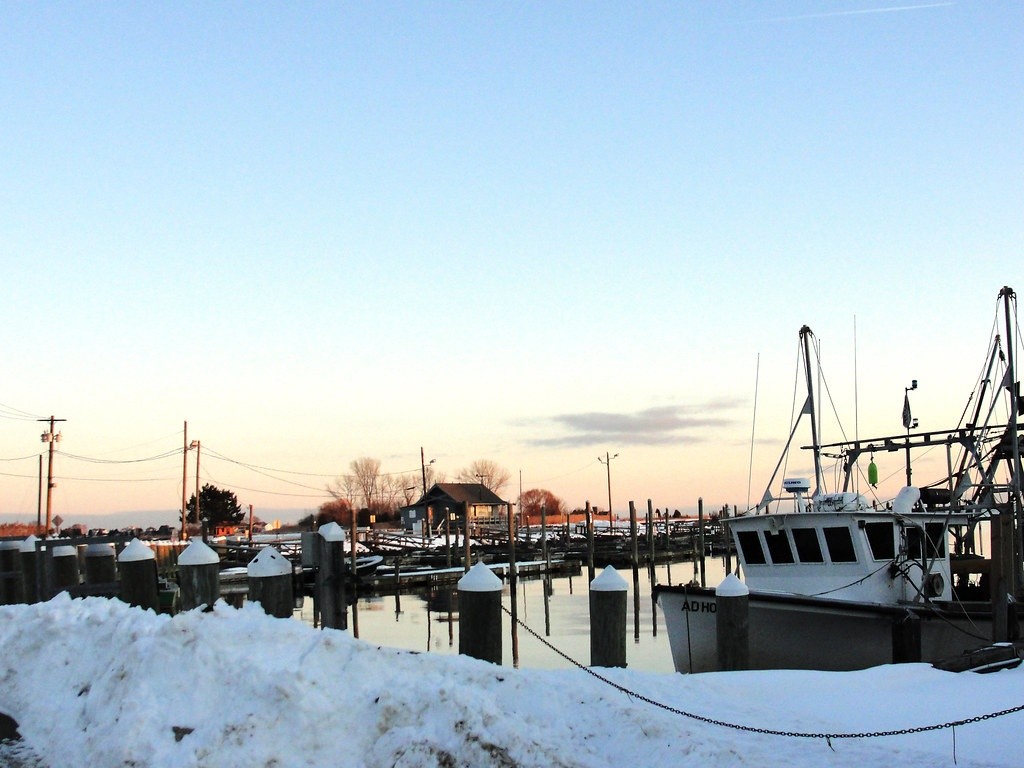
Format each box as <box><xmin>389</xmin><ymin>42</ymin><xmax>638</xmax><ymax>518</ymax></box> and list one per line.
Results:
<box><xmin>216</xmin><ymin>556</ymin><xmax>384</xmax><ymax>595</ymax></box>
<box><xmin>652</xmin><ymin>283</ymin><xmax>1024</xmax><ymax>676</ymax></box>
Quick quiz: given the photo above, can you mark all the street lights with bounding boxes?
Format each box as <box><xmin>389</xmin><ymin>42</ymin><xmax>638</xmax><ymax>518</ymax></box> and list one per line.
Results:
<box><xmin>181</xmin><ymin>441</ymin><xmax>198</xmax><ymax>541</ymax></box>
<box><xmin>41</xmin><ymin>431</ymin><xmax>61</xmax><ymax>537</ymax></box>
<box><xmin>597</xmin><ymin>453</ymin><xmax>619</xmax><ymax>533</ymax></box>
<box><xmin>421</xmin><ymin>459</ymin><xmax>437</xmax><ymax>538</ymax></box>
